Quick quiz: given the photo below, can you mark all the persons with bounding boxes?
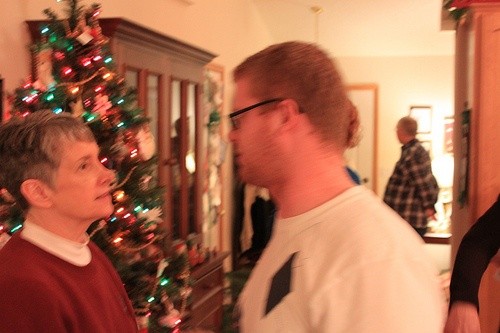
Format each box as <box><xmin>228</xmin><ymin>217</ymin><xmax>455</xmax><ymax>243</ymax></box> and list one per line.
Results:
<box><xmin>228</xmin><ymin>41</ymin><xmax>448</xmax><ymax>333</ymax></box>
<box><xmin>444</xmin><ymin>194</ymin><xmax>500</xmax><ymax>333</ymax></box>
<box><xmin>0</xmin><ymin>110</ymin><xmax>140</xmax><ymax>333</ymax></box>
<box><xmin>136</xmin><ymin>117</ymin><xmax>199</xmax><ymax>188</ymax></box>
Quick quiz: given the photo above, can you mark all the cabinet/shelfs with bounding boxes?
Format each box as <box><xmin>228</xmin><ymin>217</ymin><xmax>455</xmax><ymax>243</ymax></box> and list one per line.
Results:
<box><xmin>25</xmin><ymin>16</ymin><xmax>233</xmax><ymax>333</ymax></box>
<box><xmin>450</xmin><ymin>6</ymin><xmax>500</xmax><ymax>333</ymax></box>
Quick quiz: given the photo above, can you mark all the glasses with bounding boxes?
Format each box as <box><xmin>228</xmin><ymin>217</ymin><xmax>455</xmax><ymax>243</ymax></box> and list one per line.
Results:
<box><xmin>226</xmin><ymin>98</ymin><xmax>305</xmax><ymax>131</ymax></box>
